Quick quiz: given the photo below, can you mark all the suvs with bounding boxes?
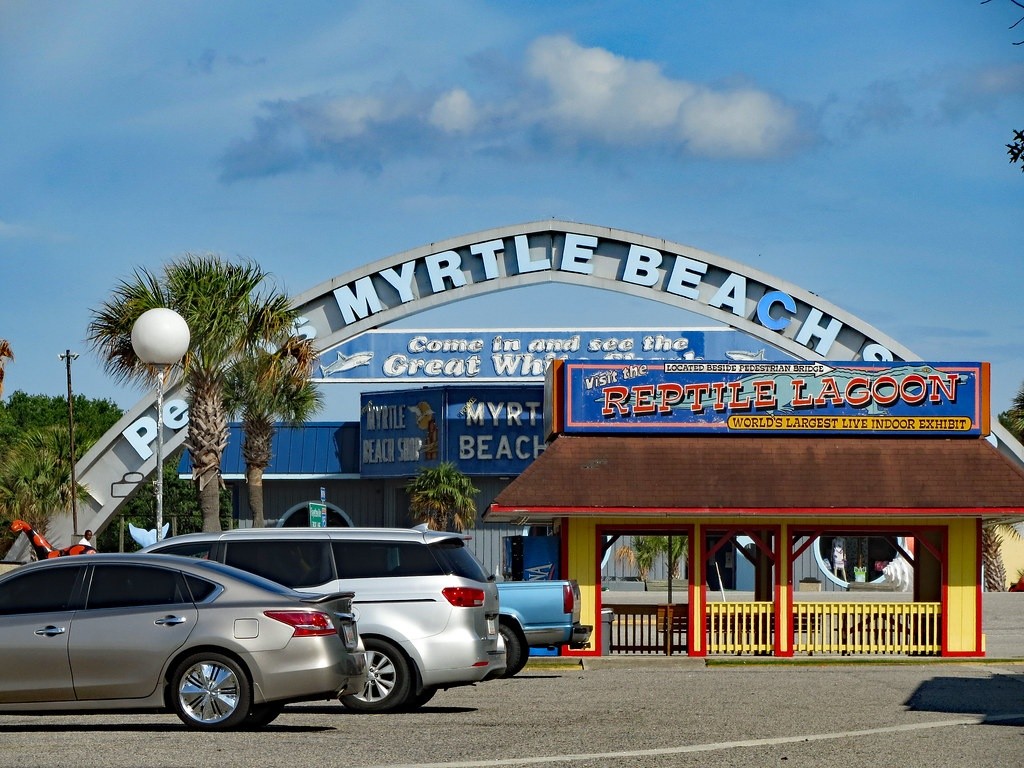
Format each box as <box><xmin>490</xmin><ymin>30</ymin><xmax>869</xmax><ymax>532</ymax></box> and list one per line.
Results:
<box><xmin>134</xmin><ymin>521</ymin><xmax>506</xmax><ymax>713</ymax></box>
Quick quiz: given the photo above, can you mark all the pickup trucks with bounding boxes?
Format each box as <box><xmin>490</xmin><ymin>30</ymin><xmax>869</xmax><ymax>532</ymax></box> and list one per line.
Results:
<box><xmin>493</xmin><ymin>579</ymin><xmax>594</xmax><ymax>678</ymax></box>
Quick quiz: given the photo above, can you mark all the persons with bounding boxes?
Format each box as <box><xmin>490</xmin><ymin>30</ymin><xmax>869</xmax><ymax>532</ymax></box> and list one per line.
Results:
<box><xmin>831</xmin><ymin>536</ymin><xmax>848</xmax><ymax>581</ymax></box>
<box><xmin>79</xmin><ymin>530</ymin><xmax>92</xmax><ymax>547</ymax></box>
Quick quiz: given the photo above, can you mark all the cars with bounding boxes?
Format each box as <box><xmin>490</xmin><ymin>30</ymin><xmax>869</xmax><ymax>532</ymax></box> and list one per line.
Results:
<box><xmin>0</xmin><ymin>553</ymin><xmax>367</xmax><ymax>730</ymax></box>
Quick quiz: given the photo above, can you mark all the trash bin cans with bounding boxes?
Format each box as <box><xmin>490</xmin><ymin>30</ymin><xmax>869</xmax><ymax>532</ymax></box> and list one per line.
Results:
<box><xmin>601</xmin><ymin>608</ymin><xmax>616</xmax><ymax>655</ymax></box>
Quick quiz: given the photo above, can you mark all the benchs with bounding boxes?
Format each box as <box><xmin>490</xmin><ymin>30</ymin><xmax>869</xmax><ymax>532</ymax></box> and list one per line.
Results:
<box><xmin>657</xmin><ymin>603</ymin><xmax>821</xmax><ymax>655</ymax></box>
<box><xmin>645</xmin><ymin>579</ymin><xmax>710</xmax><ymax>591</ymax></box>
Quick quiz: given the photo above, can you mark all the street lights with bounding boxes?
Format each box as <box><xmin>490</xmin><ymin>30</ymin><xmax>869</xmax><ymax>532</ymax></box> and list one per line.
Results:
<box><xmin>131</xmin><ymin>307</ymin><xmax>191</xmax><ymax>543</ymax></box>
<box><xmin>56</xmin><ymin>349</ymin><xmax>80</xmax><ymax>536</ymax></box>
